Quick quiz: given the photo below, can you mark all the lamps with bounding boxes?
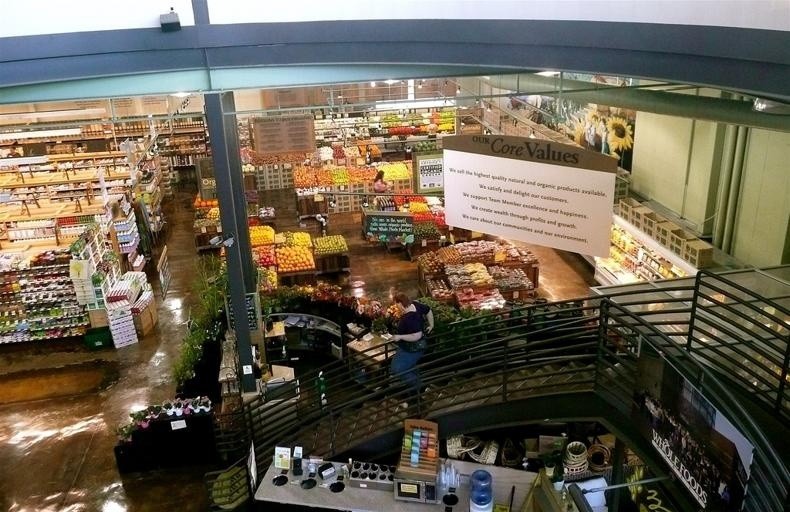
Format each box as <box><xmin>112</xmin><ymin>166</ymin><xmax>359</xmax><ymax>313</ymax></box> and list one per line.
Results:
<box><xmin>375</xmin><ymin>96</ymin><xmax>457</xmax><ymax>111</ymax></box>
<box><xmin>0</xmin><ymin>128</ymin><xmax>82</xmax><ymax>139</ymax></box>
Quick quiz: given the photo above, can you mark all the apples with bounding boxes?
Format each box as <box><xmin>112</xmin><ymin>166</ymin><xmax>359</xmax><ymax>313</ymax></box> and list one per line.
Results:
<box><xmin>251</xmin><ymin>246</ymin><xmax>316</xmax><ymax>273</ymax></box>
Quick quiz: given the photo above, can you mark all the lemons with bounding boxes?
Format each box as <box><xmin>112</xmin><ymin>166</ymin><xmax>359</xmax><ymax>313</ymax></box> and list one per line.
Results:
<box><xmin>249</xmin><ymin>225</ymin><xmax>275</xmax><ymax>246</ymax></box>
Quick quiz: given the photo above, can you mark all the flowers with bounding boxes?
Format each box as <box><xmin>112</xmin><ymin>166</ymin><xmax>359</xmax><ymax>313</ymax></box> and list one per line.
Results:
<box><xmin>267</xmin><ymin>280</ymin><xmax>403</xmax><ymax>331</ymax></box>
<box><xmin>117</xmin><ymin>396</ymin><xmax>214</xmax><ymax>443</ymax></box>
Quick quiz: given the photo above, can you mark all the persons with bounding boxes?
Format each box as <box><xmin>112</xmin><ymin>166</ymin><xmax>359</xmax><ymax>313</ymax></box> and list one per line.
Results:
<box><xmin>426</xmin><ymin>119</ymin><xmax>438</xmax><ymax>138</ymax></box>
<box><xmin>580</xmin><ymin>113</ymin><xmax>602</xmax><ymax>151</ymax></box>
<box><xmin>373</xmin><ymin>170</ymin><xmax>394</xmax><ymax>193</ymax></box>
<box><xmin>7</xmin><ymin>141</ymin><xmax>24</xmax><ymax>157</ymax></box>
<box><xmin>596</xmin><ymin>118</ymin><xmax>613</xmax><ymax>154</ymax></box>
<box><xmin>364</xmin><ymin>145</ymin><xmax>375</xmax><ymax>164</ymax></box>
<box><xmin>385</xmin><ymin>292</ymin><xmax>435</xmax><ymax>387</ymax></box>
<box><xmin>75</xmin><ymin>143</ymin><xmax>88</xmax><ymax>153</ymax></box>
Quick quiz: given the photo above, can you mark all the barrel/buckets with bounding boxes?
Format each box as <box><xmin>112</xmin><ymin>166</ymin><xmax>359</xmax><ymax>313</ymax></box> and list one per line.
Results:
<box><xmin>469</xmin><ymin>470</ymin><xmax>493</xmax><ymax>505</ymax></box>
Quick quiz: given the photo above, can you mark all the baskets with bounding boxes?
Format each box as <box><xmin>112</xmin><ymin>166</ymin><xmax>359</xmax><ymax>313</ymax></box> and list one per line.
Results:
<box><xmin>501</xmin><ymin>439</ymin><xmax>523</xmax><ymax>465</ymax></box>
<box><xmin>446</xmin><ymin>433</ymin><xmax>500</xmax><ymax>465</ymax></box>
<box><xmin>563</xmin><ymin>441</ymin><xmax>589</xmax><ymax>475</ymax></box>
<box><xmin>589</xmin><ymin>444</ymin><xmax>611</xmax><ymax>472</ymax></box>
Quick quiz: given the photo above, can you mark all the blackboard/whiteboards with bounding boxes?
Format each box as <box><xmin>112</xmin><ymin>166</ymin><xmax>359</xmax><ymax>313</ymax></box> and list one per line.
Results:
<box><xmin>366</xmin><ymin>215</ymin><xmax>413</xmax><ymax>243</ymax></box>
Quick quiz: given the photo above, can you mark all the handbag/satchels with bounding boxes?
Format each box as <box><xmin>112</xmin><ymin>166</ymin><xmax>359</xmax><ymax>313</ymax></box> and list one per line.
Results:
<box><xmin>398</xmin><ymin>338</ymin><xmax>427</xmax><ymax>352</ymax></box>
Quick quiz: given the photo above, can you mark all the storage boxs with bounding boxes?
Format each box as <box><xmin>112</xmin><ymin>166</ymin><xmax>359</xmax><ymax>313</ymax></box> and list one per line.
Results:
<box><xmin>618</xmin><ymin>196</ymin><xmax>713</xmax><ymax>270</ymax></box>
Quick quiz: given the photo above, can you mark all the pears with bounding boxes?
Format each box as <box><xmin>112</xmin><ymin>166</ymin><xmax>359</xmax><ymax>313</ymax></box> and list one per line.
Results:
<box><xmin>312</xmin><ymin>235</ymin><xmax>348</xmax><ymax>255</ymax></box>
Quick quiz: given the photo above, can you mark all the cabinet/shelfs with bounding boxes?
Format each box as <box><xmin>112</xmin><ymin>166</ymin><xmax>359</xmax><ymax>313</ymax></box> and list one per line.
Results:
<box><xmin>0</xmin><ymin>113</ymin><xmax>207</xmax><ymax>348</ymax></box>
<box><xmin>197</xmin><ymin>112</ymin><xmax>725</xmax><ymax>313</ymax></box>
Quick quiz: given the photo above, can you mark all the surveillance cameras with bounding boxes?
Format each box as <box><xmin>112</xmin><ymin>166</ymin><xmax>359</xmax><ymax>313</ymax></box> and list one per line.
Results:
<box><xmin>223</xmin><ymin>237</ymin><xmax>236</xmax><ymax>248</ymax></box>
<box><xmin>208</xmin><ymin>235</ymin><xmax>223</xmax><ymax>246</ymax></box>
